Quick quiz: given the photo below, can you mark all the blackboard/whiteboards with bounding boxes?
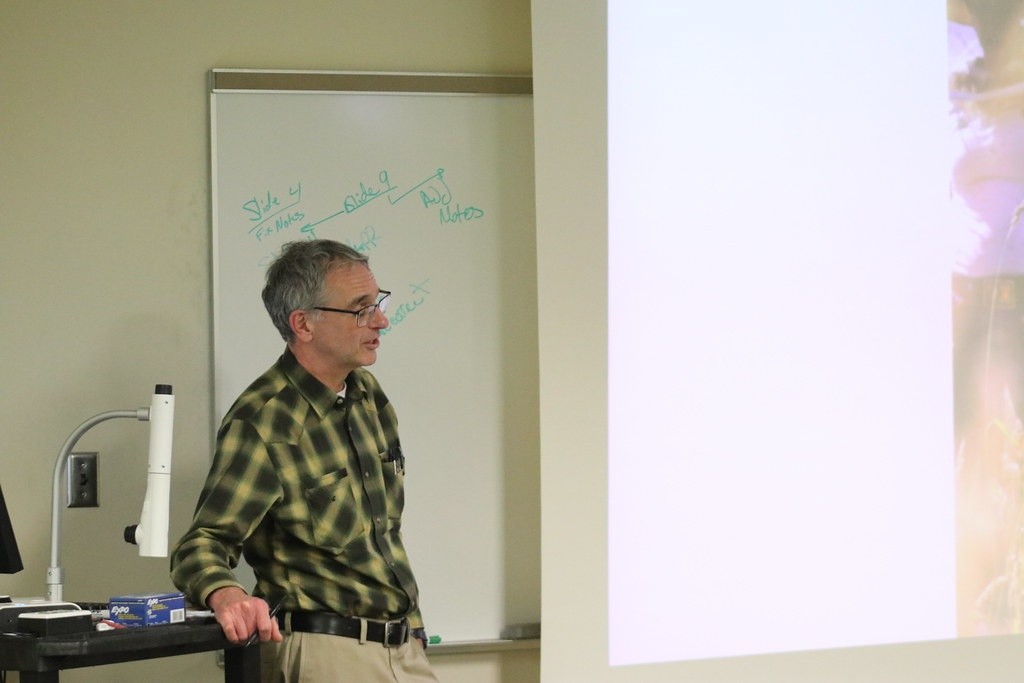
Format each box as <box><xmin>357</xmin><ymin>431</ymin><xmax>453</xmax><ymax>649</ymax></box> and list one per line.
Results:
<box><xmin>208</xmin><ymin>67</ymin><xmax>541</xmax><ymax>656</ymax></box>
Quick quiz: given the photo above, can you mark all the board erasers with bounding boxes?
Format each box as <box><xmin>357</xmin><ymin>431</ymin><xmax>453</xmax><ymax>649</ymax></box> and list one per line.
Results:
<box><xmin>499</xmin><ymin>622</ymin><xmax>542</xmax><ymax>643</ymax></box>
<box><xmin>17</xmin><ymin>610</ymin><xmax>93</xmax><ymax>637</ymax></box>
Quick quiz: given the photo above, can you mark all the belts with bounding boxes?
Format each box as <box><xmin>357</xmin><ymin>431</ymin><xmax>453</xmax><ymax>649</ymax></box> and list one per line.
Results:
<box><xmin>275</xmin><ymin>612</ymin><xmax>412</xmax><ymax>648</ymax></box>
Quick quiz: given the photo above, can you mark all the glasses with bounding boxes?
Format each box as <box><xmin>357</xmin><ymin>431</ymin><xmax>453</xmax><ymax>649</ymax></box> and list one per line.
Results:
<box><xmin>312</xmin><ymin>288</ymin><xmax>392</xmax><ymax>328</ymax></box>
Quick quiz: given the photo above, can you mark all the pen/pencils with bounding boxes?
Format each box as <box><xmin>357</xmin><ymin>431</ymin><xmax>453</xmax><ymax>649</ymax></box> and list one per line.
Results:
<box><xmin>243</xmin><ymin>595</ymin><xmax>286</xmax><ymax>648</ymax></box>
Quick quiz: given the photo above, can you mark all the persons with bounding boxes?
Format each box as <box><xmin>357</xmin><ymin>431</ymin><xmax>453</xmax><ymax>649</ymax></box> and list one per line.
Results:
<box><xmin>169</xmin><ymin>239</ymin><xmax>440</xmax><ymax>683</ymax></box>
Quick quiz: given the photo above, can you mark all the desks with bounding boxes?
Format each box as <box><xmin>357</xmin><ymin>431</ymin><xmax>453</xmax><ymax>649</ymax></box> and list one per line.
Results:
<box><xmin>0</xmin><ymin>600</ymin><xmax>266</xmax><ymax>683</ymax></box>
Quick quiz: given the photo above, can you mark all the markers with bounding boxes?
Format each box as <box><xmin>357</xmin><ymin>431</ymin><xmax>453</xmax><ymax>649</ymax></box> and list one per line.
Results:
<box><xmin>426</xmin><ymin>634</ymin><xmax>441</xmax><ymax>645</ymax></box>
